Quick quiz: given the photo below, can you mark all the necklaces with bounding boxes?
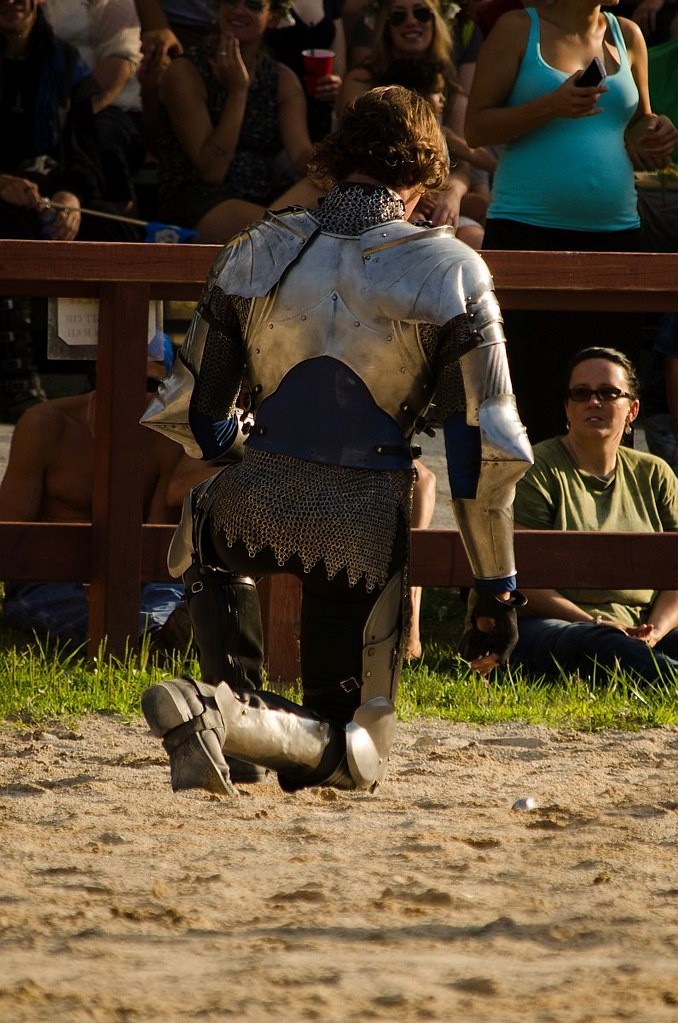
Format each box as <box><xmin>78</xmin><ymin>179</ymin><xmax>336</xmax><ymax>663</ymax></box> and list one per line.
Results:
<box><xmin>87</xmin><ymin>390</ymin><xmax>94</xmax><ymax>439</ymax></box>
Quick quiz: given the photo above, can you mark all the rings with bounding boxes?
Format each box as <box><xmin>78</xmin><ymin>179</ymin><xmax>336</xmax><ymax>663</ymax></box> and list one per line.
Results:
<box><xmin>216</xmin><ymin>50</ymin><xmax>227</xmax><ymax>56</ymax></box>
<box><xmin>448</xmin><ymin>215</ymin><xmax>454</xmax><ymax>221</ymax></box>
<box><xmin>24</xmin><ymin>185</ymin><xmax>32</xmax><ymax>192</ymax></box>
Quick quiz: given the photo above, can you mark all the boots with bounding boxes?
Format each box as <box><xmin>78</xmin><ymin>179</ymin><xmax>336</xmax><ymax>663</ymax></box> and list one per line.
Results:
<box><xmin>141</xmin><ymin>678</ymin><xmax>355</xmax><ymax>800</ymax></box>
<box><xmin>0</xmin><ymin>296</ymin><xmax>47</xmax><ymax>422</ymax></box>
<box><xmin>186</xmin><ymin>575</ymin><xmax>267</xmax><ymax>784</ymax></box>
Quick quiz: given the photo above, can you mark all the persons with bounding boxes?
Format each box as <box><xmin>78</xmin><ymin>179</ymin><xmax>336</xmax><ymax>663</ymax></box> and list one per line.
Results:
<box><xmin>140</xmin><ymin>86</ymin><xmax>533</xmax><ymax>794</ymax></box>
<box><xmin>1</xmin><ymin>0</ymin><xmax>678</xmax><ymax>688</ymax></box>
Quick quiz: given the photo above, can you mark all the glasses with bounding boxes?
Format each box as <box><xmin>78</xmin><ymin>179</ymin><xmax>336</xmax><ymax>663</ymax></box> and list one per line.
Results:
<box><xmin>145</xmin><ymin>378</ymin><xmax>162</xmax><ymax>392</ymax></box>
<box><xmin>387</xmin><ymin>7</ymin><xmax>432</xmax><ymax>27</ymax></box>
<box><xmin>568</xmin><ymin>387</ymin><xmax>634</xmax><ymax>402</ymax></box>
<box><xmin>229</xmin><ymin>0</ymin><xmax>264</xmax><ymax>11</ymax></box>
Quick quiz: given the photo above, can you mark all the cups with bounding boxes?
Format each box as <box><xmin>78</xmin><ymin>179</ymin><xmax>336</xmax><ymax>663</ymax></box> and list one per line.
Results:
<box><xmin>302</xmin><ymin>49</ymin><xmax>334</xmax><ymax>96</ymax></box>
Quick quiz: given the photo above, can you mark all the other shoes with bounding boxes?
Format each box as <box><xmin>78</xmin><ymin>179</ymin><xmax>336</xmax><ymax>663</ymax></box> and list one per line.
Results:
<box><xmin>402</xmin><ymin>640</ymin><xmax>424</xmax><ymax>671</ymax></box>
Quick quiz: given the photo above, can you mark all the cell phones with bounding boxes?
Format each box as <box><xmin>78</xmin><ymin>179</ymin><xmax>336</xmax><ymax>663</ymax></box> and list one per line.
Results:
<box><xmin>576</xmin><ymin>56</ymin><xmax>606</xmax><ymax>98</ymax></box>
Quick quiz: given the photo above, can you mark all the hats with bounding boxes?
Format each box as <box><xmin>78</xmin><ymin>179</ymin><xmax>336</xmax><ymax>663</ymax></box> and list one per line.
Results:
<box><xmin>147</xmin><ymin>330</ymin><xmax>174</xmax><ymax>377</ymax></box>
